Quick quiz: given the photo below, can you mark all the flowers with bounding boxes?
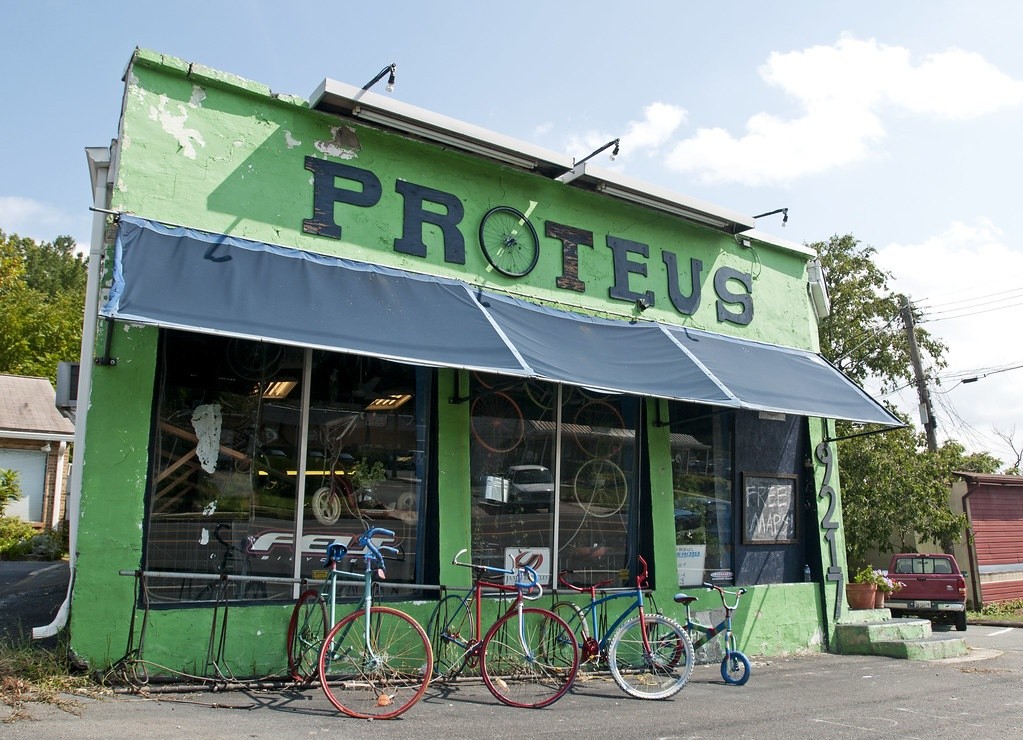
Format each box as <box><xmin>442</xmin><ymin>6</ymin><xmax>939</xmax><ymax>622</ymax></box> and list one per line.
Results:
<box><xmin>873</xmin><ymin>570</ymin><xmax>906</xmax><ymax>594</ymax></box>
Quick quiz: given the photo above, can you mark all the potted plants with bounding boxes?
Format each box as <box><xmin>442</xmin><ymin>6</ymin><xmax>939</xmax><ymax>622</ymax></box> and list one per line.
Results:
<box><xmin>846</xmin><ymin>564</ymin><xmax>876</xmax><ymax>609</ymax></box>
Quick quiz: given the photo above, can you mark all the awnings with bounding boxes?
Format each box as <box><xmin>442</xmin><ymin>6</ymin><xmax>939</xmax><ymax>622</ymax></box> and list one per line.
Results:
<box><xmin>96</xmin><ymin>216</ymin><xmax>907</xmax><ymax>441</ymax></box>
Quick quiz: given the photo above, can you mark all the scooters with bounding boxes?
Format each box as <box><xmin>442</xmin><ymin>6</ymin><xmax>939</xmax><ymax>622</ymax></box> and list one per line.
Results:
<box><xmin>312</xmin><ymin>411</ymin><xmax>418</xmax><ymax>526</ymax></box>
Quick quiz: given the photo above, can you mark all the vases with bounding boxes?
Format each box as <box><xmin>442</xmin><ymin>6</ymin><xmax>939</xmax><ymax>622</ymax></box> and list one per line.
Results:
<box><xmin>875</xmin><ymin>591</ymin><xmax>884</xmax><ymax>609</ymax></box>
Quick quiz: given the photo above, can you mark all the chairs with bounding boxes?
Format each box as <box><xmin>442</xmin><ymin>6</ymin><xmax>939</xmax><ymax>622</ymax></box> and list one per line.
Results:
<box><xmin>897</xmin><ymin>564</ymin><xmax>914</xmax><ymax>573</ymax></box>
<box><xmin>931</xmin><ymin>564</ymin><xmax>948</xmax><ymax>574</ymax></box>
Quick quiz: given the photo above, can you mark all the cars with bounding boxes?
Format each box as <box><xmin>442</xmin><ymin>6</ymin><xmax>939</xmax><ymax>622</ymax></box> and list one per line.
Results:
<box><xmin>672</xmin><ymin>448</ymin><xmax>733</xmax><ymax>480</ymax></box>
<box><xmin>503</xmin><ymin>465</ymin><xmax>554</xmax><ymax>514</ymax></box>
<box><xmin>675</xmin><ymin>497</ymin><xmax>732</xmax><ymax>532</ymax></box>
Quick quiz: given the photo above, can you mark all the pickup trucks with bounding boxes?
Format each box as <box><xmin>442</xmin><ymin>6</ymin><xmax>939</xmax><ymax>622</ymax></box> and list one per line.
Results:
<box><xmin>883</xmin><ymin>553</ymin><xmax>969</xmax><ymax>631</ymax></box>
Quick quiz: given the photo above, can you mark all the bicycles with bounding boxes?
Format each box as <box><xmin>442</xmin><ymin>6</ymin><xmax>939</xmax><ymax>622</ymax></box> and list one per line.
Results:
<box><xmin>539</xmin><ymin>555</ymin><xmax>695</xmax><ymax>701</ymax></box>
<box><xmin>427</xmin><ymin>549</ymin><xmax>579</xmax><ymax>709</ymax></box>
<box><xmin>288</xmin><ymin>527</ymin><xmax>434</xmax><ymax>720</ymax></box>
<box><xmin>642</xmin><ymin>582</ymin><xmax>751</xmax><ymax>686</ymax></box>
<box><xmin>194</xmin><ymin>523</ymin><xmax>269</xmax><ymax>600</ymax></box>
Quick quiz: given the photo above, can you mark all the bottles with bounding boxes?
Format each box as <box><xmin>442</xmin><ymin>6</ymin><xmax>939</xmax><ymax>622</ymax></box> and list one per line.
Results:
<box><xmin>803</xmin><ymin>564</ymin><xmax>811</xmax><ymax>582</ymax></box>
